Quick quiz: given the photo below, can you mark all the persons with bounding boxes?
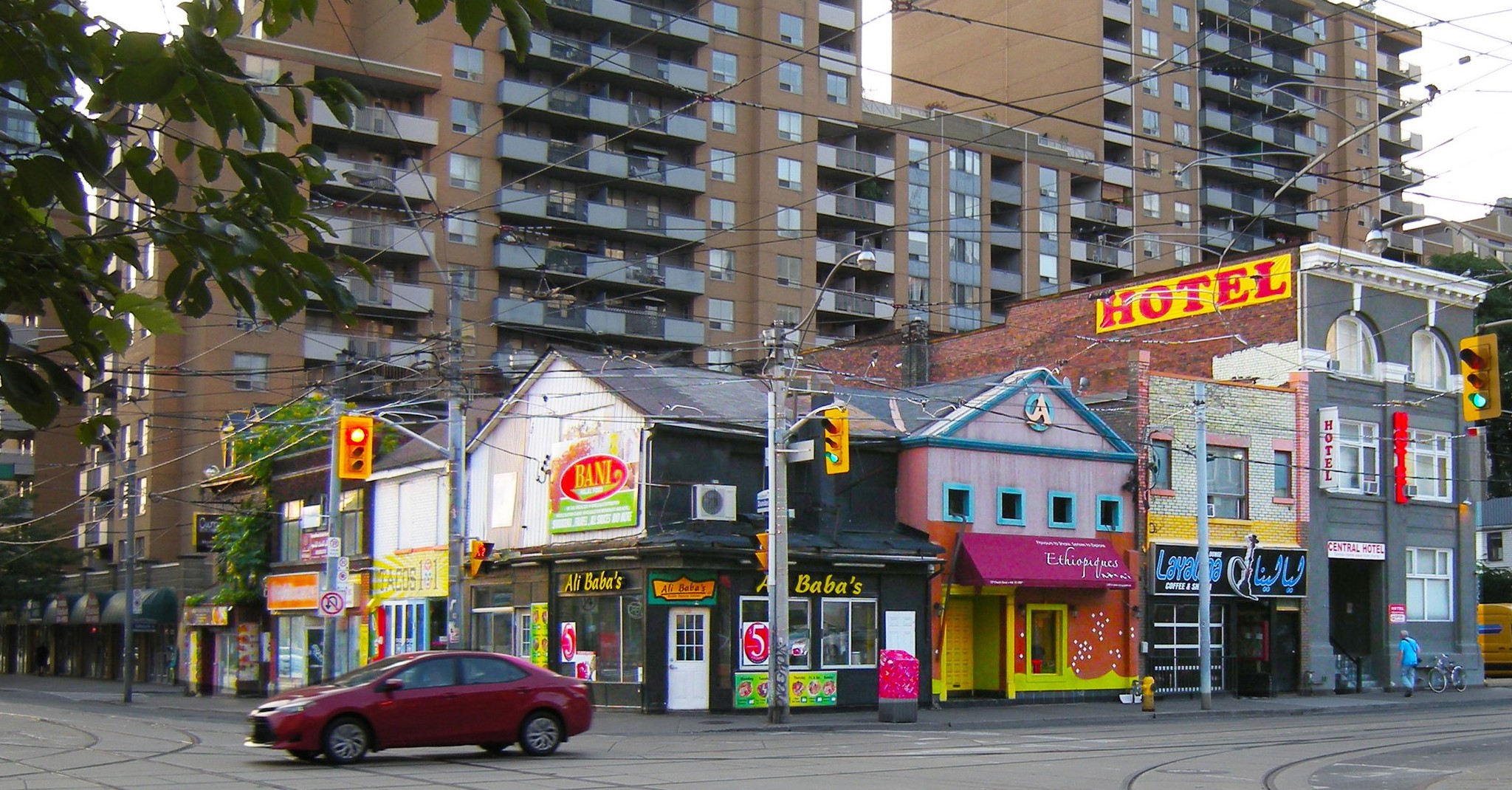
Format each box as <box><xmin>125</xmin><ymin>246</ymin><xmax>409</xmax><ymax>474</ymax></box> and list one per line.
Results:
<box><xmin>1396</xmin><ymin>630</ymin><xmax>1421</xmax><ymax>697</ymax></box>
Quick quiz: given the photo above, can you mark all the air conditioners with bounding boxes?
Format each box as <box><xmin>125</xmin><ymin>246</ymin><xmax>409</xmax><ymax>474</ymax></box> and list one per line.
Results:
<box><xmin>691</xmin><ymin>484</ymin><xmax>737</xmax><ymax>521</ymax></box>
<box><xmin>1364</xmin><ymin>480</ymin><xmax>1378</xmax><ymax>494</ymax></box>
<box><xmin>1404</xmin><ymin>372</ymin><xmax>1416</xmax><ymax>383</ymax></box>
<box><xmin>1404</xmin><ymin>485</ymin><xmax>1418</xmax><ymax>497</ymax></box>
<box><xmin>1327</xmin><ymin>359</ymin><xmax>1340</xmax><ymax>372</ymax></box>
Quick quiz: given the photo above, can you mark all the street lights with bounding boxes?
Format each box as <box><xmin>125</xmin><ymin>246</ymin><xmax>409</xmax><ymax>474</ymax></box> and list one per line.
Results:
<box><xmin>759</xmin><ymin>251</ymin><xmax>877</xmax><ymax>725</ymax></box>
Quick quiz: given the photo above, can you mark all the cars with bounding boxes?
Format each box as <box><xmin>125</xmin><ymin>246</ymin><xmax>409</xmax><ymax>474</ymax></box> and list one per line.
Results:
<box><xmin>244</xmin><ymin>649</ymin><xmax>593</xmax><ymax>765</ymax></box>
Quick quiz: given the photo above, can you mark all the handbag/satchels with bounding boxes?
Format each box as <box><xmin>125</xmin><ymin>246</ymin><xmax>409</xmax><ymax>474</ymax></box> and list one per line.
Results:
<box><xmin>1417</xmin><ymin>659</ymin><xmax>1422</xmax><ymax>663</ymax></box>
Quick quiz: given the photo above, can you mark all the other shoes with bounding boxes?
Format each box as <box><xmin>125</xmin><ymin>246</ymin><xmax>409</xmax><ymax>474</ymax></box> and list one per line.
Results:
<box><xmin>1404</xmin><ymin>691</ymin><xmax>1411</xmax><ymax>697</ymax></box>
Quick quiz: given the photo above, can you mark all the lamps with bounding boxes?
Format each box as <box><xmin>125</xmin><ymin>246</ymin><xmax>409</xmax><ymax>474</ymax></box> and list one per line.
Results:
<box><xmin>1070</xmin><ymin>605</ymin><xmax>1077</xmax><ymax>618</ymax></box>
<box><xmin>934</xmin><ymin>602</ymin><xmax>942</xmax><ymax>617</ymax></box>
<box><xmin>1017</xmin><ymin>603</ymin><xmax>1026</xmax><ymax>618</ymax></box>
<box><xmin>1123</xmin><ymin>600</ymin><xmax>1142</xmax><ymax>618</ymax></box>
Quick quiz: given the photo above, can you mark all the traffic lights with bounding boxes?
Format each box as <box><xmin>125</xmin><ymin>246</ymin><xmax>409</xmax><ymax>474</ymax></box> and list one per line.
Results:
<box><xmin>756</xmin><ymin>533</ymin><xmax>771</xmax><ymax>571</ymax></box>
<box><xmin>339</xmin><ymin>416</ymin><xmax>374</xmax><ymax>479</ymax></box>
<box><xmin>1460</xmin><ymin>333</ymin><xmax>1502</xmax><ymax>422</ymax></box>
<box><xmin>822</xmin><ymin>409</ymin><xmax>851</xmax><ymax>474</ymax></box>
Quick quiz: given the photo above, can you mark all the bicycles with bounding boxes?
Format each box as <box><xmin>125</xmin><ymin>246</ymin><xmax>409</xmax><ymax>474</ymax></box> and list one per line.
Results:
<box><xmin>1428</xmin><ymin>653</ymin><xmax>1468</xmax><ymax>693</ymax></box>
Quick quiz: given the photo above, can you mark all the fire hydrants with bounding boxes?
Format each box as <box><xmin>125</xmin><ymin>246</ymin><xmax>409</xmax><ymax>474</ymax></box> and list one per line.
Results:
<box><xmin>1140</xmin><ymin>676</ymin><xmax>1156</xmax><ymax>712</ymax></box>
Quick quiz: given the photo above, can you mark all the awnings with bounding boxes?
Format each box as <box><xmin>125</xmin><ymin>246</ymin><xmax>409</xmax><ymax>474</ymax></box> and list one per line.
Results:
<box><xmin>954</xmin><ymin>531</ymin><xmax>1137</xmax><ymax>590</ymax></box>
<box><xmin>13</xmin><ymin>587</ymin><xmax>177</xmax><ymax>631</ymax></box>
